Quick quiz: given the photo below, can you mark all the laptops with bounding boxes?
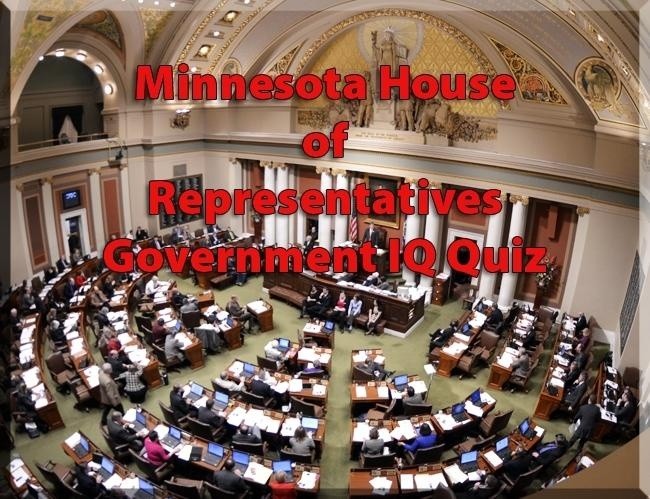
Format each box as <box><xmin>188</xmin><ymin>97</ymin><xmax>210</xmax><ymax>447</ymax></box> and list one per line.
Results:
<box><xmin>4</xmin><ymin>225</ymin><xmax>618</xmax><ymax>499</ymax></box>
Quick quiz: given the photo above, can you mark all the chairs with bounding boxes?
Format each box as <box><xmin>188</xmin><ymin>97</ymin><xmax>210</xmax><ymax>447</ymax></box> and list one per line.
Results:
<box><xmin>2</xmin><ymin>218</ymin><xmax>649</xmax><ymax>499</ymax></box>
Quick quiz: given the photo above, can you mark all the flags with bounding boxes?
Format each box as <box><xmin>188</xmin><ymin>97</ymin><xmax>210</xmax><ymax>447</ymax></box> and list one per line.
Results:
<box><xmin>351</xmin><ymin>199</ymin><xmax>359</xmax><ymax>242</ymax></box>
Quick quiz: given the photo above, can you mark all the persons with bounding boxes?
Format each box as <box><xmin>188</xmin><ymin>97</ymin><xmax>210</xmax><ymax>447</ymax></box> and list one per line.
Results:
<box><xmin>9</xmin><ymin>221</ymin><xmax>636</xmax><ymax>498</ymax></box>
<box><xmin>357</xmin><ymin>30</ymin><xmax>450</xmax><ymax>132</ymax></box>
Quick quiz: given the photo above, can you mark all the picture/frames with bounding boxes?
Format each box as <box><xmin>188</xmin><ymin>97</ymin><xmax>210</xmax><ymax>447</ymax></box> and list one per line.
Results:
<box><xmin>363</xmin><ymin>172</ymin><xmax>401</xmax><ymax>230</ymax></box>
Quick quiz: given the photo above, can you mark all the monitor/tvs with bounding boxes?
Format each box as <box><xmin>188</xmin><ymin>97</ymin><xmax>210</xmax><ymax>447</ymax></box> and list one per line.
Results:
<box><xmin>63</xmin><ymin>190</ymin><xmax>80</xmax><ymax>210</ymax></box>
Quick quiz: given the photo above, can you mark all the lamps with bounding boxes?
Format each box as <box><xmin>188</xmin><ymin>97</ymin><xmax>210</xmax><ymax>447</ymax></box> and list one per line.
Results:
<box><xmin>39</xmin><ymin>45</ymin><xmax>115</xmax><ymax>96</ymax></box>
<box><xmin>170</xmin><ymin>109</ymin><xmax>190</xmax><ymax>129</ymax></box>
